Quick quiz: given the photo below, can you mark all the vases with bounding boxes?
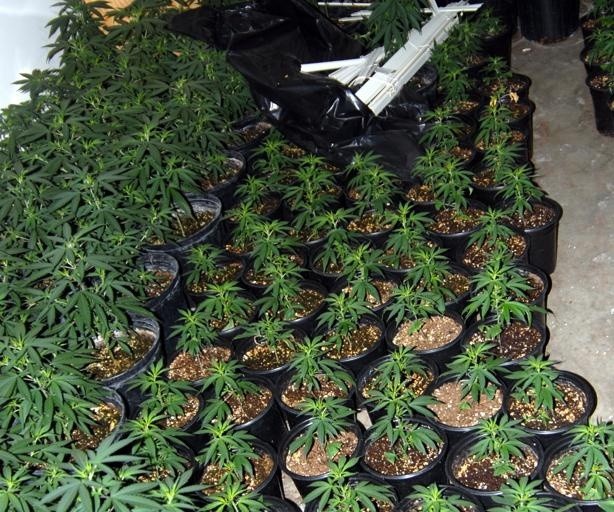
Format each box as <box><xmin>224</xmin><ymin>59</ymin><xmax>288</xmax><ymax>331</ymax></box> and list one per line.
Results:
<box><xmin>519</xmin><ymin>1</ymin><xmax>580</xmax><ymax>44</ymax></box>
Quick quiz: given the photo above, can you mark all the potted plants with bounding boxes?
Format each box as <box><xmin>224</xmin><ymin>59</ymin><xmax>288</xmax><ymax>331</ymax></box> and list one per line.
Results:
<box><xmin>539</xmin><ymin>415</ymin><xmax>614</xmax><ymax>512</ymax></box>
<box><xmin>578</xmin><ymin>1</ymin><xmax>613</xmax><ymax>134</ymax></box>
<box><xmin>1</xmin><ymin>0</ymin><xmax>562</xmax><ymax>512</ymax></box>
<box><xmin>503</xmin><ymin>354</ymin><xmax>598</xmax><ymax>451</ymax></box>
<box><xmin>485</xmin><ymin>475</ymin><xmax>583</xmax><ymax>511</ymax></box>
